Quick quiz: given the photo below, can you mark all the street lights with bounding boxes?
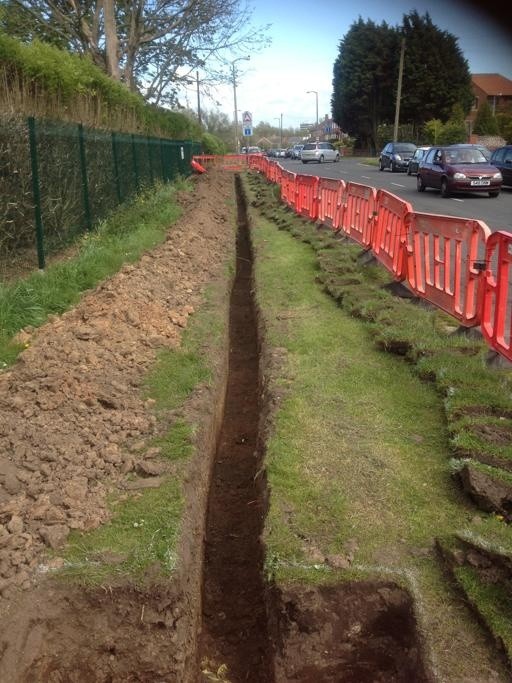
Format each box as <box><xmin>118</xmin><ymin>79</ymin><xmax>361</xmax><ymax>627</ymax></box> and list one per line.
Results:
<box><xmin>230</xmin><ymin>55</ymin><xmax>251</xmax><ymax>152</ymax></box>
<box><xmin>273</xmin><ymin>113</ymin><xmax>284</xmax><ymax>145</ymax></box>
<box><xmin>306</xmin><ymin>90</ymin><xmax>319</xmax><ymax>126</ymax></box>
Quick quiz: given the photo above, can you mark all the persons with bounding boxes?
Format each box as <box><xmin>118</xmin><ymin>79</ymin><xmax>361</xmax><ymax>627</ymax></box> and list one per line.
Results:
<box><xmin>457</xmin><ymin>148</ymin><xmax>475</xmax><ymax>162</ymax></box>
<box><xmin>438</xmin><ymin>150</ymin><xmax>454</xmax><ymax>163</ymax></box>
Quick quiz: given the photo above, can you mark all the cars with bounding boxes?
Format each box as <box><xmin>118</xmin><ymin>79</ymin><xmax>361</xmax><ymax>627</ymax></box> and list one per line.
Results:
<box><xmin>273</xmin><ymin>142</ymin><xmax>340</xmax><ymax>164</ymax></box>
<box><xmin>378</xmin><ymin>142</ymin><xmax>512</xmax><ymax>198</ymax></box>
<box><xmin>242</xmin><ymin>146</ymin><xmax>268</xmax><ymax>158</ymax></box>
